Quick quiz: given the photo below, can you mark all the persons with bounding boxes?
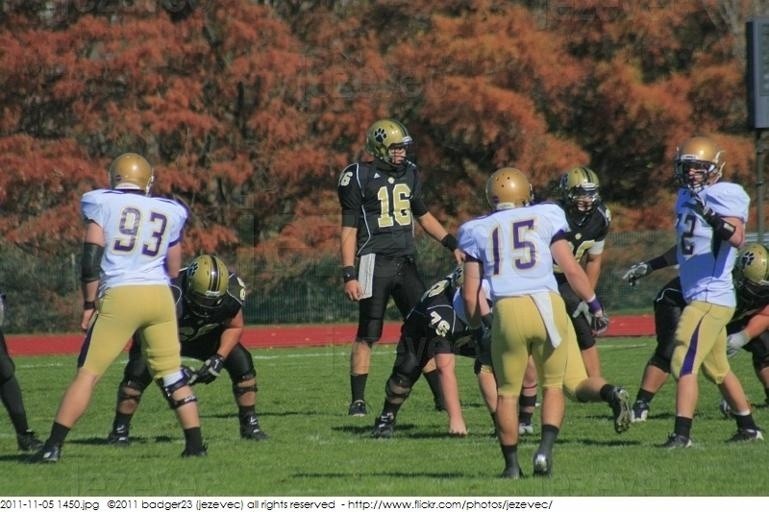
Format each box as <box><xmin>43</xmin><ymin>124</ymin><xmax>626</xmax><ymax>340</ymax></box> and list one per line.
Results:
<box><xmin>109</xmin><ymin>253</ymin><xmax>269</xmax><ymax>446</ymax></box>
<box><xmin>455</xmin><ymin>167</ymin><xmax>607</xmax><ymax>479</ymax></box>
<box><xmin>622</xmin><ymin>135</ymin><xmax>764</xmax><ymax>448</ymax></box>
<box><xmin>629</xmin><ymin>242</ymin><xmax>769</xmax><ymax>426</ymax></box>
<box><xmin>372</xmin><ymin>268</ymin><xmax>475</xmax><ymax>436</ymax></box>
<box><xmin>31</xmin><ymin>152</ymin><xmax>207</xmax><ymax>464</ymax></box>
<box><xmin>0</xmin><ymin>293</ymin><xmax>45</xmax><ymax>451</ymax></box>
<box><xmin>336</xmin><ymin>119</ymin><xmax>465</xmax><ymax>415</ymax></box>
<box><xmin>542</xmin><ymin>166</ymin><xmax>612</xmax><ymax>379</ymax></box>
<box><xmin>452</xmin><ymin>278</ymin><xmax>631</xmax><ymax>436</ymax></box>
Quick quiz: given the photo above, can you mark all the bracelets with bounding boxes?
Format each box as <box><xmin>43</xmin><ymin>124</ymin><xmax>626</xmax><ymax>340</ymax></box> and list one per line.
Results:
<box><xmin>523</xmin><ymin>385</ymin><xmax>537</xmax><ymax>389</ymax></box>
<box><xmin>442</xmin><ymin>233</ymin><xmax>458</xmax><ymax>250</ymax></box>
<box><xmin>648</xmin><ymin>254</ymin><xmax>668</xmax><ymax>271</ymax></box>
<box><xmin>469</xmin><ymin>326</ymin><xmax>482</xmax><ymax>337</ymax></box>
<box><xmin>588</xmin><ymin>295</ymin><xmax>602</xmax><ymax>313</ymax></box>
<box><xmin>520</xmin><ymin>394</ymin><xmax>536</xmax><ymax>406</ymax></box>
<box><xmin>716</xmin><ymin>219</ymin><xmax>735</xmax><ymax>241</ymax></box>
<box><xmin>342</xmin><ymin>266</ymin><xmax>356</xmax><ymax>282</ymax></box>
<box><xmin>82</xmin><ymin>300</ymin><xmax>96</xmax><ymax>310</ymax></box>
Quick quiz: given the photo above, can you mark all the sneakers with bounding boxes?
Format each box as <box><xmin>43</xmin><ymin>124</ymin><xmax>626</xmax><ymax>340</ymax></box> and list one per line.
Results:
<box><xmin>501</xmin><ymin>467</ymin><xmax>521</xmax><ymax>480</ymax></box>
<box><xmin>534</xmin><ymin>454</ymin><xmax>551</xmax><ymax>477</ymax></box>
<box><xmin>631</xmin><ymin>400</ymin><xmax>649</xmax><ymax>422</ymax></box>
<box><xmin>518</xmin><ymin>421</ymin><xmax>533</xmax><ymax>435</ymax></box>
<box><xmin>349</xmin><ymin>400</ymin><xmax>366</xmax><ymax>417</ymax></box>
<box><xmin>17</xmin><ymin>431</ymin><xmax>44</xmax><ymax>451</ymax></box>
<box><xmin>108</xmin><ymin>423</ymin><xmax>129</xmax><ymax>445</ymax></box>
<box><xmin>240</xmin><ymin>424</ymin><xmax>271</xmax><ymax>441</ymax></box>
<box><xmin>181</xmin><ymin>447</ymin><xmax>208</xmax><ymax>459</ymax></box>
<box><xmin>29</xmin><ymin>443</ymin><xmax>60</xmax><ymax>463</ymax></box>
<box><xmin>657</xmin><ymin>433</ymin><xmax>693</xmax><ymax>447</ymax></box>
<box><xmin>724</xmin><ymin>428</ymin><xmax>765</xmax><ymax>444</ymax></box>
<box><xmin>608</xmin><ymin>386</ymin><xmax>630</xmax><ymax>433</ymax></box>
<box><xmin>372</xmin><ymin>414</ymin><xmax>396</xmax><ymax>437</ymax></box>
<box><xmin>719</xmin><ymin>399</ymin><xmax>732</xmax><ymax>419</ymax></box>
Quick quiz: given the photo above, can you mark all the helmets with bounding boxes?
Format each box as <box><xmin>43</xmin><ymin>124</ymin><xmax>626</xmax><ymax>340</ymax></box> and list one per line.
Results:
<box><xmin>486</xmin><ymin>167</ymin><xmax>534</xmax><ymax>210</ymax></box>
<box><xmin>109</xmin><ymin>154</ymin><xmax>154</xmax><ymax>194</ymax></box>
<box><xmin>186</xmin><ymin>254</ymin><xmax>230</xmax><ymax>317</ymax></box>
<box><xmin>451</xmin><ymin>262</ymin><xmax>465</xmax><ymax>295</ymax></box>
<box><xmin>672</xmin><ymin>136</ymin><xmax>727</xmax><ymax>190</ymax></box>
<box><xmin>559</xmin><ymin>167</ymin><xmax>602</xmax><ymax>225</ymax></box>
<box><xmin>366</xmin><ymin>119</ymin><xmax>413</xmax><ymax>172</ymax></box>
<box><xmin>736</xmin><ymin>242</ymin><xmax>769</xmax><ymax>289</ymax></box>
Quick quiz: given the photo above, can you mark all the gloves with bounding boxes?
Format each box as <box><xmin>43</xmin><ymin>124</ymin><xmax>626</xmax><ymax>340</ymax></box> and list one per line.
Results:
<box><xmin>182</xmin><ymin>366</ymin><xmax>198</xmax><ymax>385</ymax></box>
<box><xmin>198</xmin><ymin>353</ymin><xmax>225</xmax><ymax>385</ymax></box>
<box><xmin>684</xmin><ymin>184</ymin><xmax>719</xmax><ymax>225</ymax></box>
<box><xmin>468</xmin><ymin>325</ymin><xmax>492</xmax><ymax>365</ymax></box>
<box><xmin>573</xmin><ymin>296</ymin><xmax>609</xmax><ymax>337</ymax></box>
<box><xmin>725</xmin><ymin>332</ymin><xmax>748</xmax><ymax>357</ymax></box>
<box><xmin>622</xmin><ymin>262</ymin><xmax>654</xmax><ymax>287</ymax></box>
